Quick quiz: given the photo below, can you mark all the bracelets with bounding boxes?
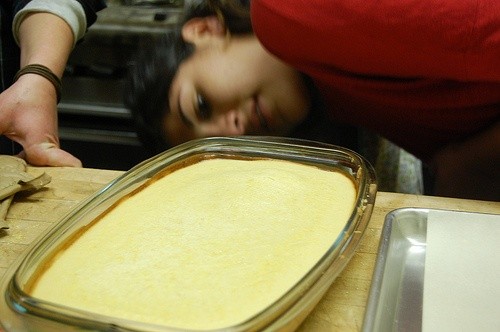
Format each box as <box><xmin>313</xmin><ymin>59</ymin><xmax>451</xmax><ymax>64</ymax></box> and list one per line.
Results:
<box><xmin>14</xmin><ymin>63</ymin><xmax>63</xmax><ymax>106</ymax></box>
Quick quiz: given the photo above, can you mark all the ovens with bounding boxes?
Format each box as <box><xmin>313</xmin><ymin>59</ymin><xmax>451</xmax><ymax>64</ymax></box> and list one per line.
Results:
<box><xmin>56</xmin><ymin>22</ymin><xmax>171</xmax><ymax>121</ymax></box>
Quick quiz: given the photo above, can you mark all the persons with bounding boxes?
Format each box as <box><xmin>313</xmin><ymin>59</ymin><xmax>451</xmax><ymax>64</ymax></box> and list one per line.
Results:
<box><xmin>1</xmin><ymin>0</ymin><xmax>111</xmax><ymax>169</ymax></box>
<box><xmin>119</xmin><ymin>0</ymin><xmax>500</xmax><ymax>203</ymax></box>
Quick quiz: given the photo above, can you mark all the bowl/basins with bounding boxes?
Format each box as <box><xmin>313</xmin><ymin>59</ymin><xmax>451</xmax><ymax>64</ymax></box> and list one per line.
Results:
<box><xmin>0</xmin><ymin>136</ymin><xmax>378</xmax><ymax>332</ymax></box>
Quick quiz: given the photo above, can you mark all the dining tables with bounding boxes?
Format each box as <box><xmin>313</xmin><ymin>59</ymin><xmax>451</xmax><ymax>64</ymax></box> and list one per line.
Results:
<box><xmin>0</xmin><ymin>167</ymin><xmax>500</xmax><ymax>332</ymax></box>
